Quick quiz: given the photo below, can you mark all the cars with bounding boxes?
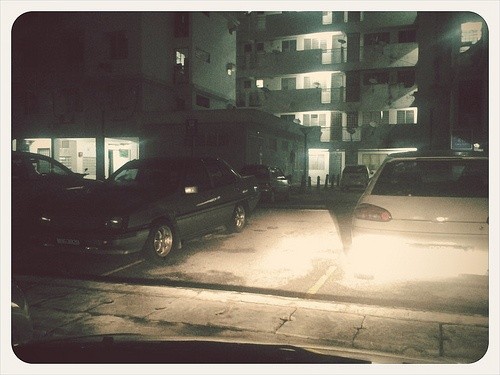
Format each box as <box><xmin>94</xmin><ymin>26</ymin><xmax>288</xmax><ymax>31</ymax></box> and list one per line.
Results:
<box><xmin>11</xmin><ymin>151</ymin><xmax>105</xmax><ymax>217</ymax></box>
<box><xmin>350</xmin><ymin>150</ymin><xmax>489</xmax><ymax>284</ymax></box>
<box><xmin>236</xmin><ymin>164</ymin><xmax>292</xmax><ymax>203</ymax></box>
<box><xmin>34</xmin><ymin>156</ymin><xmax>263</xmax><ymax>262</ymax></box>
<box><xmin>341</xmin><ymin>165</ymin><xmax>374</xmax><ymax>189</ymax></box>
